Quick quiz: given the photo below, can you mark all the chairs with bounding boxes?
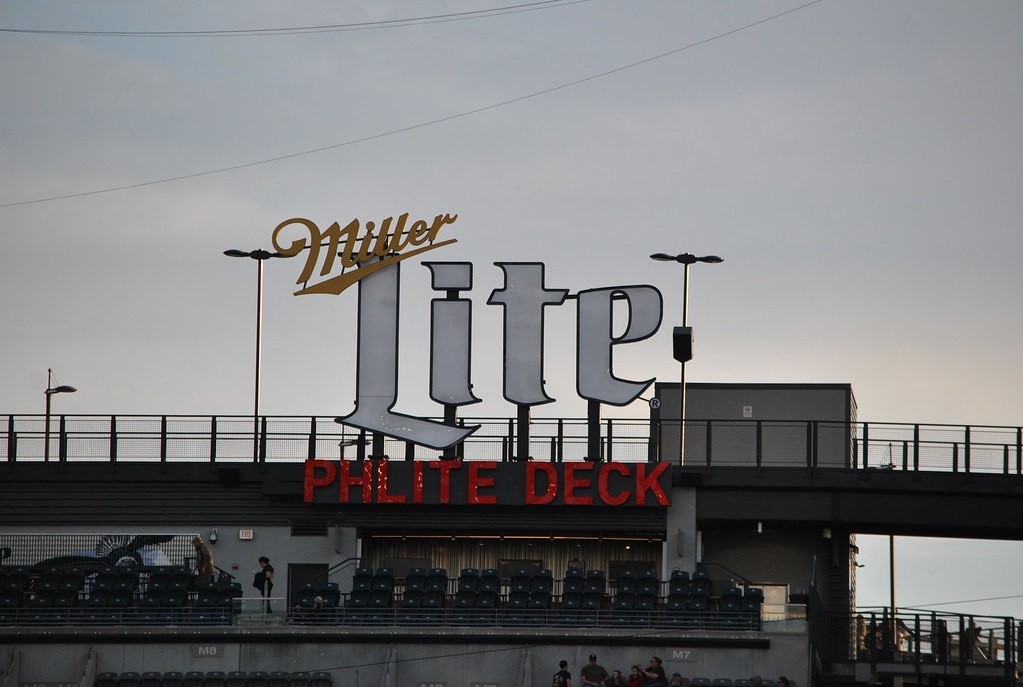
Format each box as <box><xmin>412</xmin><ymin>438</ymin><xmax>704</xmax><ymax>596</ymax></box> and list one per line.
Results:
<box><xmin>0</xmin><ymin>561</ymin><xmax>242</xmax><ymax>626</ymax></box>
<box><xmin>670</xmin><ymin>678</ymin><xmax>796</xmax><ymax>687</ymax></box>
<box><xmin>287</xmin><ymin>569</ymin><xmax>764</xmax><ymax>631</ymax></box>
<box><xmin>93</xmin><ymin>671</ymin><xmax>333</xmax><ymax>687</ymax></box>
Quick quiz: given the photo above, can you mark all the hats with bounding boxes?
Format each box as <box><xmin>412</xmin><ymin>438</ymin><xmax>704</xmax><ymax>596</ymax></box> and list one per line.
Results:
<box><xmin>589</xmin><ymin>654</ymin><xmax>596</xmax><ymax>661</ymax></box>
<box><xmin>190</xmin><ymin>537</ymin><xmax>201</xmax><ymax>543</ymax></box>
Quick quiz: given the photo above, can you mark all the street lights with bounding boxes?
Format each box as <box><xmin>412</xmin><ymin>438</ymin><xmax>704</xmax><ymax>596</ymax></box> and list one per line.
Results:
<box><xmin>44</xmin><ymin>367</ymin><xmax>77</xmax><ymax>462</ymax></box>
<box><xmin>650</xmin><ymin>251</ymin><xmax>725</xmax><ymax>468</ymax></box>
<box><xmin>222</xmin><ymin>247</ymin><xmax>295</xmax><ymax>461</ymax></box>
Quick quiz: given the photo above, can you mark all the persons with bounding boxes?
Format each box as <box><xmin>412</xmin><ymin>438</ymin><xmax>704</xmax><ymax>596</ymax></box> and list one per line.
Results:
<box><xmin>581</xmin><ymin>654</ymin><xmax>609</xmax><ymax>687</ymax></box>
<box><xmin>310</xmin><ymin>596</ymin><xmax>334</xmax><ymax>625</ymax></box>
<box><xmin>966</xmin><ymin>623</ymin><xmax>982</xmax><ymax>664</ymax></box>
<box><xmin>552</xmin><ymin>660</ymin><xmax>571</xmax><ymax>687</ymax></box>
<box><xmin>750</xmin><ymin>674</ymin><xmax>767</xmax><ymax>687</ymax></box>
<box><xmin>856</xmin><ymin>615</ymin><xmax>866</xmax><ymax>662</ymax></box>
<box><xmin>778</xmin><ymin>676</ymin><xmax>790</xmax><ymax>687</ymax></box>
<box><xmin>608</xmin><ymin>670</ymin><xmax>625</xmax><ymax>687</ymax></box>
<box><xmin>627</xmin><ymin>666</ymin><xmax>644</xmax><ymax>687</ymax></box>
<box><xmin>252</xmin><ymin>557</ymin><xmax>274</xmax><ymax>614</ymax></box>
<box><xmin>286</xmin><ymin>601</ymin><xmax>305</xmax><ymax>625</ymax></box>
<box><xmin>638</xmin><ymin>656</ymin><xmax>668</xmax><ymax>687</ymax></box>
<box><xmin>190</xmin><ymin>537</ymin><xmax>212</xmax><ymax>606</ymax></box>
<box><xmin>670</xmin><ymin>672</ymin><xmax>684</xmax><ymax>687</ymax></box>
<box><xmin>936</xmin><ymin>619</ymin><xmax>953</xmax><ymax>664</ymax></box>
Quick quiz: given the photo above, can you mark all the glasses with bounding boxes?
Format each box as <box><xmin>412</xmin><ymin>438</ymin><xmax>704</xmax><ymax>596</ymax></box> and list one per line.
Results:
<box><xmin>650</xmin><ymin>660</ymin><xmax>653</xmax><ymax>662</ymax></box>
<box><xmin>750</xmin><ymin>678</ymin><xmax>754</xmax><ymax>681</ymax></box>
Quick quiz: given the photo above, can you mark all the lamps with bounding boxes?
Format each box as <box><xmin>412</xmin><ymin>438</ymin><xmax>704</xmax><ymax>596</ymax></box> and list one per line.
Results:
<box><xmin>208</xmin><ymin>528</ymin><xmax>218</xmax><ymax>545</ymax></box>
